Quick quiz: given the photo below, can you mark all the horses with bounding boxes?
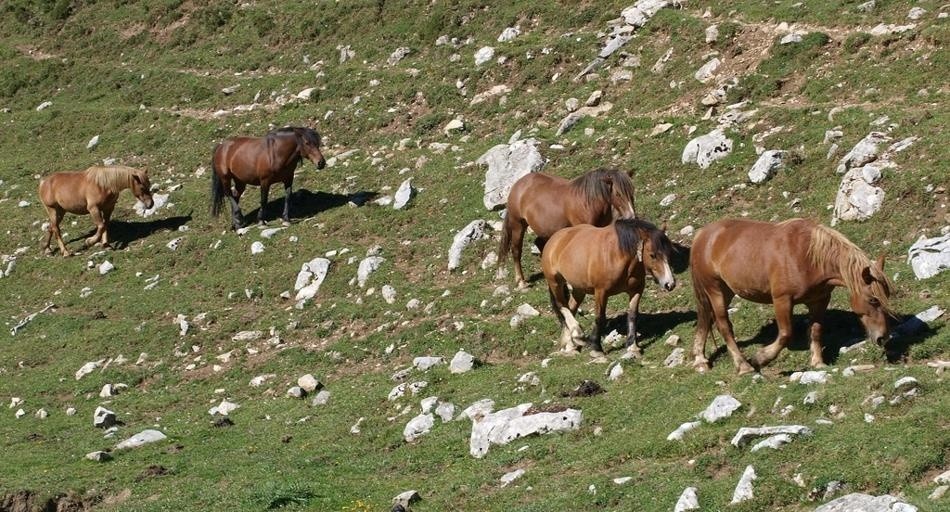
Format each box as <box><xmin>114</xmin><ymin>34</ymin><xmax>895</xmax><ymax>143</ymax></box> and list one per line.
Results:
<box><xmin>208</xmin><ymin>126</ymin><xmax>327</xmax><ymax>235</ymax></box>
<box><xmin>539</xmin><ymin>216</ymin><xmax>677</xmax><ymax>359</ymax></box>
<box><xmin>38</xmin><ymin>163</ymin><xmax>155</xmax><ymax>259</ymax></box>
<box><xmin>494</xmin><ymin>164</ymin><xmax>638</xmax><ymax>290</ymax></box>
<box><xmin>687</xmin><ymin>216</ymin><xmax>901</xmax><ymax>377</ymax></box>
